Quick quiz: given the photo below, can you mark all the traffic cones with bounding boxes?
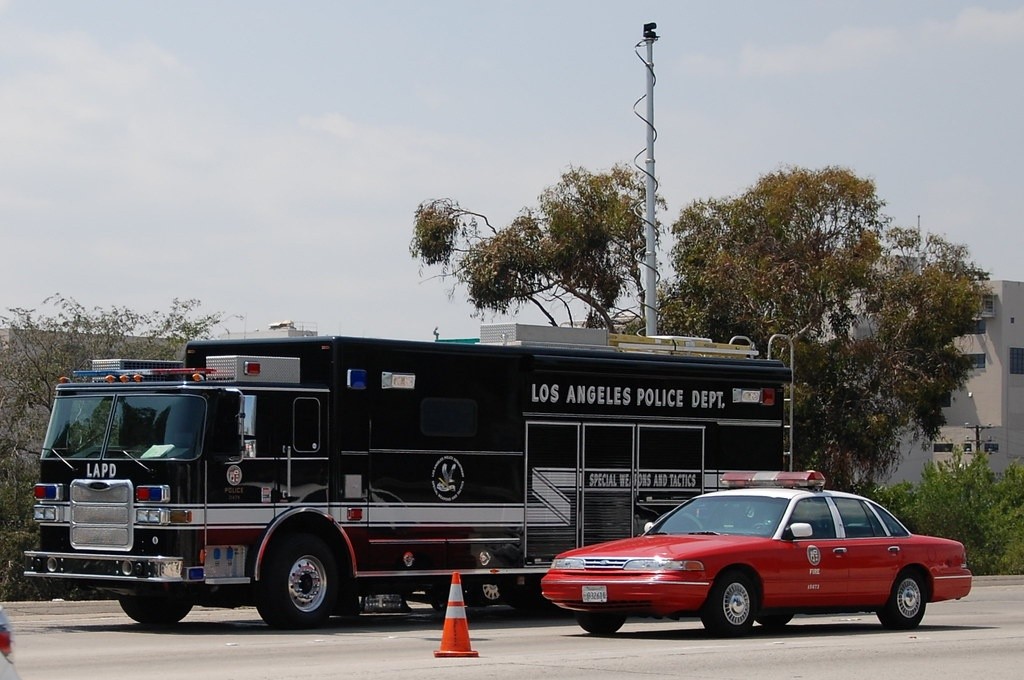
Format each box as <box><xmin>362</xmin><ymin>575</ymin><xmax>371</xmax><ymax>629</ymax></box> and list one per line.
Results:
<box><xmin>434</xmin><ymin>574</ymin><xmax>480</xmax><ymax>659</ymax></box>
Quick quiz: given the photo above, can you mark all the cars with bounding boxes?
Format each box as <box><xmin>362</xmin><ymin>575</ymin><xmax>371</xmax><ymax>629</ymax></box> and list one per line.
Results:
<box><xmin>539</xmin><ymin>469</ymin><xmax>973</xmax><ymax>636</ymax></box>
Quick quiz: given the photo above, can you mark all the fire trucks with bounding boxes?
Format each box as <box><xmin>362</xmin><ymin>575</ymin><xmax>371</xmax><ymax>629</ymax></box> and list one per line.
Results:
<box><xmin>23</xmin><ymin>325</ymin><xmax>800</xmax><ymax>629</ymax></box>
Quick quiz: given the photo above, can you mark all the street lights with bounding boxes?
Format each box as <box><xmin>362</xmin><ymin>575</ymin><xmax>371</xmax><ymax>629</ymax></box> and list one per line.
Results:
<box><xmin>634</xmin><ymin>23</ymin><xmax>661</xmax><ymax>342</ymax></box>
